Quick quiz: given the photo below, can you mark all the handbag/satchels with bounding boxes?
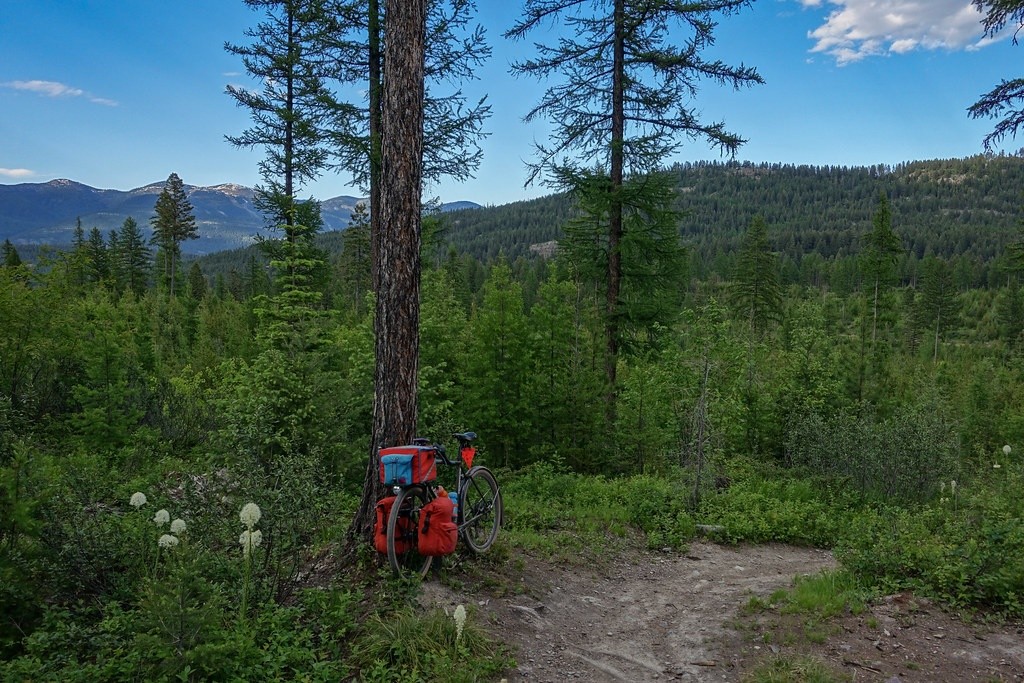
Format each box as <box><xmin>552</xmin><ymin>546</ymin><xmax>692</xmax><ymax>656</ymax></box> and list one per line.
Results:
<box><xmin>418</xmin><ymin>497</ymin><xmax>458</xmax><ymax>556</ymax></box>
<box><xmin>374</xmin><ymin>496</ymin><xmax>413</xmax><ymax>554</ymax></box>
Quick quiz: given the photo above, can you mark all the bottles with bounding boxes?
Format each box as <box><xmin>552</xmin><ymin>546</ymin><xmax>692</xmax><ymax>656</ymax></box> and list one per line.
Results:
<box><xmin>435</xmin><ymin>485</ymin><xmax>450</xmax><ymax>499</ymax></box>
<box><xmin>448</xmin><ymin>488</ymin><xmax>458</xmax><ymax>517</ymax></box>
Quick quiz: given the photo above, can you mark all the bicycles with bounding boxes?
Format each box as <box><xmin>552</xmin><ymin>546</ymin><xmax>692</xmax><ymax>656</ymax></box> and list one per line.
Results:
<box><xmin>387</xmin><ymin>430</ymin><xmax>504</xmax><ymax>588</ymax></box>
